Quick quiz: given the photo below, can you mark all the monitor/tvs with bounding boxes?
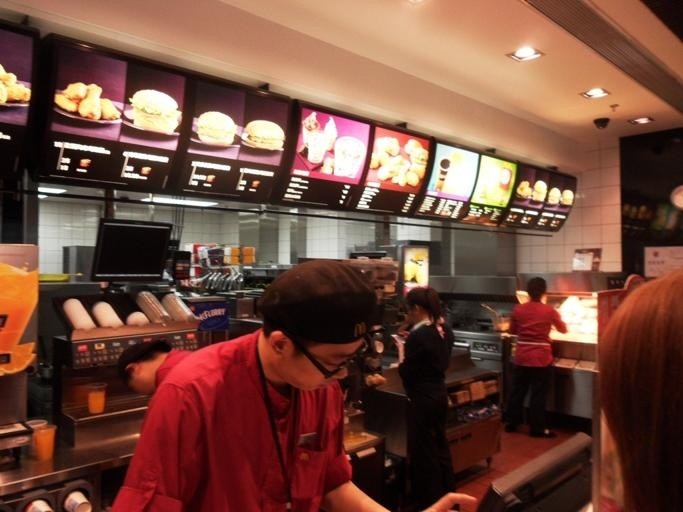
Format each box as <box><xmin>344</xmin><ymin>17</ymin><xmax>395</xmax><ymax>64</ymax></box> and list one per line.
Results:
<box><xmin>90</xmin><ymin>217</ymin><xmax>172</xmax><ymax>282</ymax></box>
<box><xmin>475</xmin><ymin>432</ymin><xmax>594</xmax><ymax>512</ymax></box>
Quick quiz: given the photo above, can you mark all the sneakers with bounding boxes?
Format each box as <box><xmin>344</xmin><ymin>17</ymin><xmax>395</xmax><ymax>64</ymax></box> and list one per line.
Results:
<box><xmin>504</xmin><ymin>423</ymin><xmax>557</xmax><ymax>438</ymax></box>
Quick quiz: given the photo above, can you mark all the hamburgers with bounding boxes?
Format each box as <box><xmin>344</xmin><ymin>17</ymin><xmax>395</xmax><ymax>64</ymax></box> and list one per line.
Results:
<box><xmin>131</xmin><ymin>89</ymin><xmax>179</xmax><ymax>133</ymax></box>
<box><xmin>246</xmin><ymin>119</ymin><xmax>285</xmax><ymax>150</ymax></box>
<box><xmin>405</xmin><ymin>140</ymin><xmax>428</xmax><ymax>167</ymax></box>
<box><xmin>197</xmin><ymin>111</ymin><xmax>236</xmax><ymax>146</ymax></box>
<box><xmin>532</xmin><ymin>181</ymin><xmax>574</xmax><ymax>206</ymax></box>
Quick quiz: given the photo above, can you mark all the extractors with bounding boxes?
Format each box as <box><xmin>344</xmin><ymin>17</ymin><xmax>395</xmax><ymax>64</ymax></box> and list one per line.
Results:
<box><xmin>425</xmin><ymin>224</ymin><xmax>519</xmax><ymax>305</ymax></box>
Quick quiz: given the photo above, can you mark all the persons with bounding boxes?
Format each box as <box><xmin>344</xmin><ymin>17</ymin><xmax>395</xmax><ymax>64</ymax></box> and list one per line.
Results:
<box><xmin>110</xmin><ymin>261</ymin><xmax>479</xmax><ymax>511</ymax></box>
<box><xmin>594</xmin><ymin>266</ymin><xmax>682</xmax><ymax>512</ymax></box>
<box><xmin>502</xmin><ymin>276</ymin><xmax>566</xmax><ymax>437</ymax></box>
<box><xmin>389</xmin><ymin>287</ymin><xmax>459</xmax><ymax>511</ymax></box>
<box><xmin>116</xmin><ymin>339</ymin><xmax>197</xmax><ymax>427</ymax></box>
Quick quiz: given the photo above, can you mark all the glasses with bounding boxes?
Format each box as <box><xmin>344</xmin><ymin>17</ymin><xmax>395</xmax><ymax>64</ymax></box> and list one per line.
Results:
<box><xmin>292</xmin><ymin>338</ymin><xmax>369</xmax><ymax>379</ymax></box>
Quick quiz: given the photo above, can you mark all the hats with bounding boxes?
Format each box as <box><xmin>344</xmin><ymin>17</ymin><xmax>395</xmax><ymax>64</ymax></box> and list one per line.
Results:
<box><xmin>257</xmin><ymin>258</ymin><xmax>373</xmax><ymax>345</ymax></box>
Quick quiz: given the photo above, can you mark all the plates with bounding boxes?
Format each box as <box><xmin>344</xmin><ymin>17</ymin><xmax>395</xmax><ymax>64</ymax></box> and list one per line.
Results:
<box><xmin>0</xmin><ymin>100</ymin><xmax>30</xmax><ymax>109</ymax></box>
<box><xmin>190</xmin><ymin>133</ymin><xmax>241</xmax><ymax>148</ymax></box>
<box><xmin>122</xmin><ymin>119</ymin><xmax>180</xmax><ymax>137</ymax></box>
<box><xmin>53</xmin><ymin>105</ymin><xmax>122</xmax><ymax>126</ymax></box>
<box><xmin>239</xmin><ymin>134</ymin><xmax>282</xmax><ymax>151</ymax></box>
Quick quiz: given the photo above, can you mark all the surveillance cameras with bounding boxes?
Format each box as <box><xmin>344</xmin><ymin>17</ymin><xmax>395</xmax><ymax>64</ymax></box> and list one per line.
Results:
<box><xmin>594</xmin><ymin>117</ymin><xmax>609</xmax><ymax>129</ymax></box>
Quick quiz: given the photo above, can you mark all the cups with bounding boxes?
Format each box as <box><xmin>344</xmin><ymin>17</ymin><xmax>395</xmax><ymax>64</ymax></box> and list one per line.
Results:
<box><xmin>31</xmin><ymin>424</ymin><xmax>57</xmax><ymax>463</ymax></box>
<box><xmin>197</xmin><ymin>245</ymin><xmax>254</xmax><ymax>267</ymax></box>
<box><xmin>307</xmin><ymin>129</ymin><xmax>328</xmax><ymax>165</ymax></box>
<box><xmin>63</xmin><ymin>490</ymin><xmax>91</xmax><ymax>511</ymax></box>
<box><xmin>24</xmin><ymin>419</ymin><xmax>48</xmax><ymax>459</ymax></box>
<box><xmin>23</xmin><ymin>498</ymin><xmax>52</xmax><ymax>512</ymax></box>
<box><xmin>436</xmin><ymin>159</ymin><xmax>450</xmax><ymax>191</ymax></box>
<box><xmin>61</xmin><ymin>289</ymin><xmax>195</xmax><ymax>331</ymax></box>
<box><xmin>85</xmin><ymin>382</ymin><xmax>106</xmax><ymax>414</ymax></box>
<box><xmin>332</xmin><ymin>137</ymin><xmax>365</xmax><ymax>179</ymax></box>
<box><xmin>303</xmin><ymin>121</ymin><xmax>320</xmax><ymax>147</ymax></box>
